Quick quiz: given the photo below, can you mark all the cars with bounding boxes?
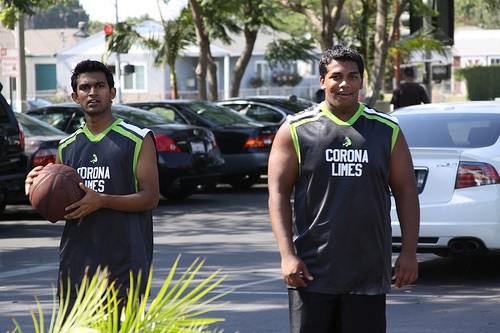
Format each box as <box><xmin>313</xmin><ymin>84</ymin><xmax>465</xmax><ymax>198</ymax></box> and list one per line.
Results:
<box><xmin>216</xmin><ymin>94</ymin><xmax>319</xmax><ymax>130</ymax></box>
<box><xmin>117</xmin><ymin>100</ymin><xmax>279</xmax><ymax>188</ymax></box>
<box><xmin>13</xmin><ymin>112</ymin><xmax>71</xmax><ymax>174</ymax></box>
<box><xmin>387</xmin><ymin>100</ymin><xmax>500</xmax><ymax>272</ymax></box>
<box><xmin>25</xmin><ymin>104</ymin><xmax>233</xmax><ymax>196</ymax></box>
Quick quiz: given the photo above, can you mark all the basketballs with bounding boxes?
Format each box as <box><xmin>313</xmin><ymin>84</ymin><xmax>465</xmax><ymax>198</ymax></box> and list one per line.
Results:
<box><xmin>28</xmin><ymin>164</ymin><xmax>85</xmax><ymax>222</ymax></box>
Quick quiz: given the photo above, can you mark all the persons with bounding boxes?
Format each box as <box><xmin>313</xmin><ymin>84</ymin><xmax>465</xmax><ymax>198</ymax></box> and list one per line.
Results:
<box><xmin>389</xmin><ymin>67</ymin><xmax>431</xmax><ymax>111</ymax></box>
<box><xmin>268</xmin><ymin>45</ymin><xmax>421</xmax><ymax>333</ymax></box>
<box><xmin>25</xmin><ymin>60</ymin><xmax>158</xmax><ymax>329</ymax></box>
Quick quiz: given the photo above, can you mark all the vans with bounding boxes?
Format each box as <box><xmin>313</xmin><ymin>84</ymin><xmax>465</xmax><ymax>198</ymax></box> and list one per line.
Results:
<box><xmin>0</xmin><ymin>82</ymin><xmax>26</xmax><ymax>216</ymax></box>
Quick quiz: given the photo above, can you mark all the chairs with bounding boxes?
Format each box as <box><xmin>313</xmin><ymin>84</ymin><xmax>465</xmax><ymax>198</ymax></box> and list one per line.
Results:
<box><xmin>465</xmin><ymin>126</ymin><xmax>495</xmax><ymax>147</ymax></box>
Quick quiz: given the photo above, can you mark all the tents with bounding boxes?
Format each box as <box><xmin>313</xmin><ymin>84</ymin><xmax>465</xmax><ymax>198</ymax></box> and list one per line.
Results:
<box><xmin>56</xmin><ymin>18</ymin><xmax>230</xmax><ymax>101</ymax></box>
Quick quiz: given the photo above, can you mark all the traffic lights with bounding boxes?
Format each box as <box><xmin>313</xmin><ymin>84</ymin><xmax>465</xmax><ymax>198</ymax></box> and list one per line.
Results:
<box><xmin>426</xmin><ymin>0</ymin><xmax>455</xmax><ymax>46</ymax></box>
<box><xmin>399</xmin><ymin>0</ymin><xmax>423</xmax><ymax>36</ymax></box>
